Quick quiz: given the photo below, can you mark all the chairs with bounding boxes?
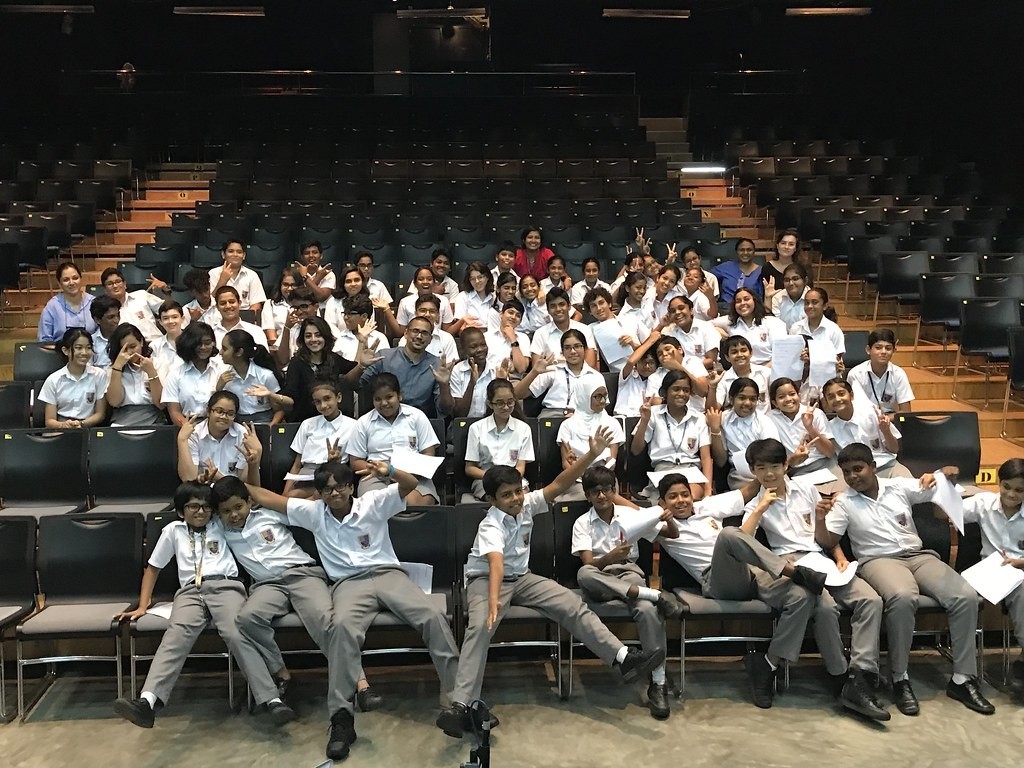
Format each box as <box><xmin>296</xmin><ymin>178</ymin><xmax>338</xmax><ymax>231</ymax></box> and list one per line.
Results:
<box><xmin>0</xmin><ymin>93</ymin><xmax>1024</xmax><ymax>723</ymax></box>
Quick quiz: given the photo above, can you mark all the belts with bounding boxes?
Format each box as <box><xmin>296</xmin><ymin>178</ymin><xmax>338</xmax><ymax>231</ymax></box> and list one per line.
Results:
<box><xmin>189</xmin><ymin>575</ymin><xmax>244</xmax><ymax>584</ymax></box>
<box><xmin>290</xmin><ymin>563</ymin><xmax>316</xmax><ymax>568</ymax></box>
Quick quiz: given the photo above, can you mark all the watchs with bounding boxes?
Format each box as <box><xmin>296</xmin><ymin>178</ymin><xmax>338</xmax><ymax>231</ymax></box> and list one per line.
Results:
<box><xmin>710</xmin><ymin>431</ymin><xmax>721</xmax><ymax>436</ymax></box>
<box><xmin>511</xmin><ymin>342</ymin><xmax>519</xmax><ymax>346</ymax></box>
<box><xmin>162</xmin><ymin>285</ymin><xmax>173</xmax><ymax>295</ymax></box>
<box><xmin>80</xmin><ymin>421</ymin><xmax>84</xmax><ymax>427</ymax></box>
<box><xmin>302</xmin><ymin>275</ymin><xmax>309</xmax><ymax>283</ymax></box>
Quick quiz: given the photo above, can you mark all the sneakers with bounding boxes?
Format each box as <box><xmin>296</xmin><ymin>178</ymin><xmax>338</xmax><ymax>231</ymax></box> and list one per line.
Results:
<box><xmin>655</xmin><ymin>589</ymin><xmax>682</xmax><ymax>621</ymax></box>
<box><xmin>113</xmin><ymin>647</ymin><xmax>1024</xmax><ymax>759</ymax></box>
<box><xmin>792</xmin><ymin>565</ymin><xmax>827</xmax><ymax>595</ymax></box>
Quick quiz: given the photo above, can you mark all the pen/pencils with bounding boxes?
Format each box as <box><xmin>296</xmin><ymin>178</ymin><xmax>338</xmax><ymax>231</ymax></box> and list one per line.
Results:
<box><xmin>620</xmin><ymin>529</ymin><xmax>623</xmax><ymax>546</ymax></box>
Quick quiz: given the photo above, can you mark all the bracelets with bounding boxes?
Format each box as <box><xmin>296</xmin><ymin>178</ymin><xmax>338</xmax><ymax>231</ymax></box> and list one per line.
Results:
<box><xmin>386</xmin><ymin>463</ymin><xmax>394</xmax><ymax>481</ymax></box>
<box><xmin>382</xmin><ymin>307</ymin><xmax>390</xmax><ymax>313</ymax></box>
<box><xmin>358</xmin><ymin>364</ymin><xmax>366</xmax><ymax>370</ymax></box>
<box><xmin>284</xmin><ymin>326</ymin><xmax>290</xmax><ymax>330</ymax></box>
<box><xmin>148</xmin><ymin>374</ymin><xmax>159</xmax><ymax>382</ymax></box>
<box><xmin>112</xmin><ymin>368</ymin><xmax>122</xmax><ymax>372</ymax></box>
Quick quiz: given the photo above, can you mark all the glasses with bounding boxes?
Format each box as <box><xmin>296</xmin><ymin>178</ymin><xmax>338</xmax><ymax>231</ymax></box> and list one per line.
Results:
<box><xmin>183</xmin><ymin>503</ymin><xmax>213</xmax><ymax>512</ymax></box>
<box><xmin>417</xmin><ymin>308</ymin><xmax>439</xmax><ymax>315</ymax></box>
<box><xmin>319</xmin><ymin>484</ymin><xmax>350</xmax><ymax>495</ymax></box>
<box><xmin>341</xmin><ymin>311</ymin><xmax>361</xmax><ymax>317</ymax></box>
<box><xmin>407</xmin><ymin>328</ymin><xmax>433</xmax><ymax>337</ymax></box>
<box><xmin>469</xmin><ymin>275</ymin><xmax>485</xmax><ymax>282</ymax></box>
<box><xmin>588</xmin><ymin>486</ymin><xmax>615</xmax><ymax>497</ymax></box>
<box><xmin>281</xmin><ymin>281</ymin><xmax>300</xmax><ymax>289</ymax></box>
<box><xmin>490</xmin><ymin>398</ymin><xmax>516</xmax><ymax>408</ymax></box>
<box><xmin>359</xmin><ymin>264</ymin><xmax>373</xmax><ymax>269</ymax></box>
<box><xmin>562</xmin><ymin>344</ymin><xmax>583</xmax><ymax>352</ymax></box>
<box><xmin>105</xmin><ymin>279</ymin><xmax>123</xmax><ymax>287</ymax></box>
<box><xmin>638</xmin><ymin>359</ymin><xmax>658</xmax><ymax>367</ymax></box>
<box><xmin>592</xmin><ymin>392</ymin><xmax>608</xmax><ymax>400</ymax></box>
<box><xmin>210</xmin><ymin>408</ymin><xmax>236</xmax><ymax>420</ymax></box>
<box><xmin>784</xmin><ymin>275</ymin><xmax>803</xmax><ymax>283</ymax></box>
<box><xmin>312</xmin><ymin>394</ymin><xmax>337</xmax><ymax>406</ymax></box>
<box><xmin>290</xmin><ymin>302</ymin><xmax>314</xmax><ymax>312</ymax></box>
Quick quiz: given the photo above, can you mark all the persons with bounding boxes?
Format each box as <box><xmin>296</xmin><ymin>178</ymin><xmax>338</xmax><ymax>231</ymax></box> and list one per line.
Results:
<box><xmin>561</xmin><ymin>441</ymin><xmax>827</xmax><ymax>708</ymax></box>
<box><xmin>435</xmin><ymin>425</ymin><xmax>666</xmax><ymax>737</ymax></box>
<box><xmin>739</xmin><ymin>438</ymin><xmax>891</xmax><ymax>720</ymax></box>
<box><xmin>345</xmin><ymin>372</ymin><xmax>441</xmax><ymax>506</ymax></box>
<box><xmin>465</xmin><ymin>370</ymin><xmax>917</xmax><ymax>502</ymax></box>
<box><xmin>571</xmin><ymin>466</ymin><xmax>683</xmax><ymax>710</ymax></box>
<box><xmin>178</xmin><ymin>390</ymin><xmax>262</xmax><ymax>487</ymax></box>
<box><xmin>919</xmin><ymin>457</ymin><xmax>1024</xmax><ymax>691</ymax></box>
<box><xmin>114</xmin><ymin>480</ymin><xmax>296</xmax><ymax>729</ymax></box>
<box><xmin>815</xmin><ymin>443</ymin><xmax>996</xmax><ymax>715</ymax></box>
<box><xmin>202</xmin><ymin>456</ymin><xmax>500</xmax><ymax>761</ymax></box>
<box><xmin>198</xmin><ymin>467</ymin><xmax>382</xmax><ymax>712</ymax></box>
<box><xmin>36</xmin><ymin>224</ymin><xmax>915</xmax><ymax>450</ymax></box>
<box><xmin>281</xmin><ymin>383</ymin><xmax>357</xmax><ymax>501</ymax></box>
